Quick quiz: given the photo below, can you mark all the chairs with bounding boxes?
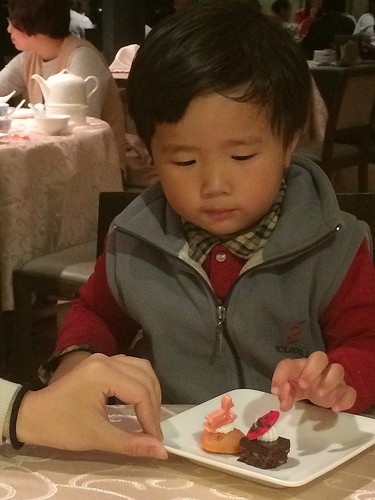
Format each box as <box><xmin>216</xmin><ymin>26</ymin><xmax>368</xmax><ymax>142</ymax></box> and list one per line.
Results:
<box><xmin>12</xmin><ymin>239</ymin><xmax>97</xmax><ymax>384</ymax></box>
<box><xmin>298</xmin><ymin>65</ymin><xmax>375</xmax><ymax>193</ymax></box>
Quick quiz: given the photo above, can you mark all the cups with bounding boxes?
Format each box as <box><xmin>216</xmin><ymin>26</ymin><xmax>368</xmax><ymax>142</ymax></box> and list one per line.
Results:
<box><xmin>44</xmin><ymin>104</ymin><xmax>87</xmax><ymax>129</ymax></box>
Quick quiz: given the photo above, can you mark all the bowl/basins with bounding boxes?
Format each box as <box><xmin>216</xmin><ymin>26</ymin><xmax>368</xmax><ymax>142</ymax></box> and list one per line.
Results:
<box><xmin>0</xmin><ymin>120</ymin><xmax>12</xmax><ymax>132</ymax></box>
<box><xmin>34</xmin><ymin>115</ymin><xmax>71</xmax><ymax>135</ymax></box>
<box><xmin>0</xmin><ymin>103</ymin><xmax>8</xmax><ymax>116</ymax></box>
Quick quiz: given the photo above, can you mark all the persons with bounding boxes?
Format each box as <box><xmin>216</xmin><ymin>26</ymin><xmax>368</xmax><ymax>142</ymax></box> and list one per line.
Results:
<box><xmin>36</xmin><ymin>0</ymin><xmax>375</xmax><ymax>415</ymax></box>
<box><xmin>0</xmin><ymin>352</ymin><xmax>168</xmax><ymax>460</ymax></box>
<box><xmin>0</xmin><ymin>0</ymin><xmax>127</xmax><ymax>192</ymax></box>
<box><xmin>271</xmin><ymin>0</ymin><xmax>375</xmax><ymax>60</ymax></box>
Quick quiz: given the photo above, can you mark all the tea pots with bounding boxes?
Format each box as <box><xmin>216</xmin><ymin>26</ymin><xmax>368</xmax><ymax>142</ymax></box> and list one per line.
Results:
<box><xmin>31</xmin><ymin>69</ymin><xmax>99</xmax><ymax>104</ymax></box>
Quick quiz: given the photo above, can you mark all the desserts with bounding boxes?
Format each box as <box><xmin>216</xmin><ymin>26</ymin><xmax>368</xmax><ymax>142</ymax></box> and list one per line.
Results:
<box><xmin>199</xmin><ymin>394</ymin><xmax>245</xmax><ymax>453</ymax></box>
<box><xmin>237</xmin><ymin>410</ymin><xmax>290</xmax><ymax>470</ymax></box>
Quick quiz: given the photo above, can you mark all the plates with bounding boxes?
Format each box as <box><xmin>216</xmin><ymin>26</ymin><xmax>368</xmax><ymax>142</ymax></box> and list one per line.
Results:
<box><xmin>8</xmin><ymin>107</ymin><xmax>34</xmax><ymax>119</ymax></box>
<box><xmin>136</xmin><ymin>389</ymin><xmax>375</xmax><ymax>489</ymax></box>
<box><xmin>0</xmin><ymin>133</ymin><xmax>8</xmax><ymax>139</ymax></box>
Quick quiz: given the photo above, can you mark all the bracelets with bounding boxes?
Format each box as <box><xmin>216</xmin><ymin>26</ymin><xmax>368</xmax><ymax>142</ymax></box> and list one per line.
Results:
<box><xmin>9</xmin><ymin>382</ymin><xmax>34</xmax><ymax>450</ymax></box>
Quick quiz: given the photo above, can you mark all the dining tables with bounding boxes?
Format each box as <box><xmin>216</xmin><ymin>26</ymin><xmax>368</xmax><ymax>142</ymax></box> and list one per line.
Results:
<box><xmin>1</xmin><ymin>404</ymin><xmax>374</xmax><ymax>500</ymax></box>
<box><xmin>0</xmin><ymin>107</ymin><xmax>124</xmax><ymax>311</ymax></box>
<box><xmin>304</xmin><ymin>59</ymin><xmax>375</xmax><ymax>193</ymax></box>
